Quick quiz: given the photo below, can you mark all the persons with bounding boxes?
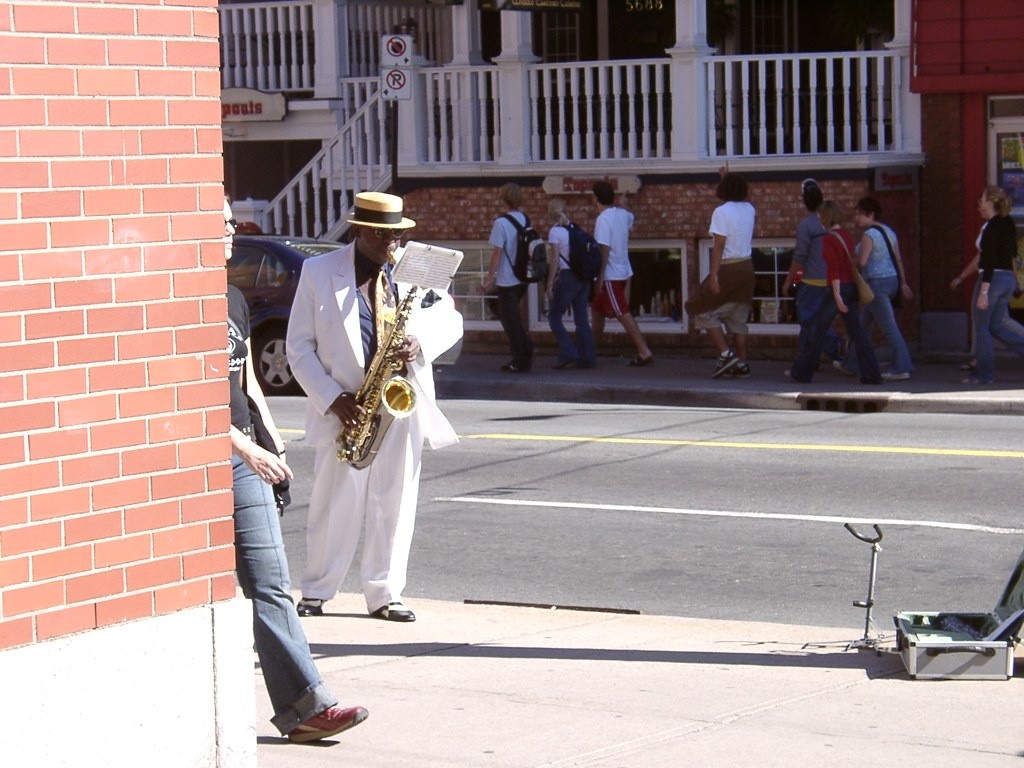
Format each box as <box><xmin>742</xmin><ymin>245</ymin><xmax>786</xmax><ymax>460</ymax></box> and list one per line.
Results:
<box><xmin>683</xmin><ymin>159</ymin><xmax>758</xmax><ymax>379</ymax></box>
<box><xmin>589</xmin><ymin>180</ymin><xmax>654</xmax><ymax>365</ymax></box>
<box><xmin>225</xmin><ymin>194</ymin><xmax>370</xmax><ymax>744</ymax></box>
<box><xmin>481</xmin><ymin>183</ymin><xmax>533</xmax><ymax>372</ymax></box>
<box><xmin>948</xmin><ymin>186</ymin><xmax>1024</xmax><ymax>385</ymax></box>
<box><xmin>284</xmin><ymin>191</ymin><xmax>465</xmax><ymax>624</ymax></box>
<box><xmin>546</xmin><ymin>198</ymin><xmax>597</xmax><ymax>369</ymax></box>
<box><xmin>781</xmin><ymin>178</ymin><xmax>916</xmax><ymax>382</ymax></box>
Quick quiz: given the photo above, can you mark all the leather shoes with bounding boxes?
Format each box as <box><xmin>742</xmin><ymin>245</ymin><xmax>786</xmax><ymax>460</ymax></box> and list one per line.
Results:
<box><xmin>296</xmin><ymin>596</ymin><xmax>327</xmax><ymax>616</ymax></box>
<box><xmin>375</xmin><ymin>603</ymin><xmax>415</xmax><ymax>621</ymax></box>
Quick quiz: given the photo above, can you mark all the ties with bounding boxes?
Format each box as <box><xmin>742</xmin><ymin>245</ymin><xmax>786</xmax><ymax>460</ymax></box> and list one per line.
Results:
<box><xmin>373</xmin><ymin>265</ymin><xmax>399</xmax><ymax>349</ymax></box>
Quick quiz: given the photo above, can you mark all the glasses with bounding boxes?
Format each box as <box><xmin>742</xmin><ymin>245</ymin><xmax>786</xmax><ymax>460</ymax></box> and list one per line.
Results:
<box><xmin>224</xmin><ymin>219</ymin><xmax>237</xmax><ymax>229</ymax></box>
<box><xmin>374</xmin><ymin>227</ymin><xmax>405</xmax><ymax>238</ymax></box>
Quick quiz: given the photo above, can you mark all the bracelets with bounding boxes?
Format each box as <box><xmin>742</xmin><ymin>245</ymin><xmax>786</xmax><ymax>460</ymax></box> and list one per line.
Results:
<box><xmin>279</xmin><ymin>449</ymin><xmax>285</xmax><ymax>455</ymax></box>
<box><xmin>959</xmin><ymin>276</ymin><xmax>964</xmax><ymax>281</ymax></box>
<box><xmin>979</xmin><ymin>291</ymin><xmax>988</xmax><ymax>295</ymax></box>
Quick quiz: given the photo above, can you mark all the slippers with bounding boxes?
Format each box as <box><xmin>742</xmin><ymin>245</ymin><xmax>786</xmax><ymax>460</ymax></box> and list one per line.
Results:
<box><xmin>628</xmin><ymin>354</ymin><xmax>656</xmax><ymax>365</ymax></box>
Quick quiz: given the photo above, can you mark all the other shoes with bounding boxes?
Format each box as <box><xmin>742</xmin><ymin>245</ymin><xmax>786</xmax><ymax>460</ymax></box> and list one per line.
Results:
<box><xmin>882</xmin><ymin>371</ymin><xmax>910</xmax><ymax>380</ymax></box>
<box><xmin>959</xmin><ymin>362</ymin><xmax>976</xmax><ymax>370</ymax></box>
<box><xmin>961</xmin><ymin>377</ymin><xmax>994</xmax><ymax>385</ymax></box>
<box><xmin>553</xmin><ymin>356</ymin><xmax>575</xmax><ymax>369</ymax></box>
<box><xmin>500</xmin><ymin>362</ymin><xmax>532</xmax><ymax>373</ymax></box>
<box><xmin>833</xmin><ymin>360</ymin><xmax>855</xmax><ymax>376</ymax></box>
<box><xmin>860</xmin><ymin>377</ymin><xmax>882</xmax><ymax>384</ymax></box>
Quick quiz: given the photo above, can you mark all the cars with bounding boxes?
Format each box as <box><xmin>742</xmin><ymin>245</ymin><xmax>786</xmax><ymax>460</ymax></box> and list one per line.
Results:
<box><xmin>224</xmin><ymin>235</ymin><xmax>351</xmax><ymax>399</ymax></box>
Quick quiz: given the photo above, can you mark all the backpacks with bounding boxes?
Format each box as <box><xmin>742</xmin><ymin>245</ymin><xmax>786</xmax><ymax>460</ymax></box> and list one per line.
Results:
<box><xmin>499</xmin><ymin>211</ymin><xmax>547</xmax><ymax>282</ymax></box>
<box><xmin>553</xmin><ymin>222</ymin><xmax>602</xmax><ymax>279</ymax></box>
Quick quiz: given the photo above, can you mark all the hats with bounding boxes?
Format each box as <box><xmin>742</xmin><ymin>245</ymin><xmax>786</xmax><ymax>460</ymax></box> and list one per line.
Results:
<box><xmin>348</xmin><ymin>192</ymin><xmax>416</xmax><ymax>229</ymax></box>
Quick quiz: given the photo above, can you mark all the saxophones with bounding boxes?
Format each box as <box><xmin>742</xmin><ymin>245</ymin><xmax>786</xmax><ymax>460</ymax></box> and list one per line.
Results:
<box><xmin>332</xmin><ymin>250</ymin><xmax>417</xmax><ymax>471</ymax></box>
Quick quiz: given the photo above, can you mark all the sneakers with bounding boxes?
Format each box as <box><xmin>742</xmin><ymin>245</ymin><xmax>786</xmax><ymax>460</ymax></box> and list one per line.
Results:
<box><xmin>711</xmin><ymin>349</ymin><xmax>740</xmax><ymax>378</ymax></box>
<box><xmin>289</xmin><ymin>706</ymin><xmax>369</xmax><ymax>741</ymax></box>
<box><xmin>722</xmin><ymin>363</ymin><xmax>751</xmax><ymax>378</ymax></box>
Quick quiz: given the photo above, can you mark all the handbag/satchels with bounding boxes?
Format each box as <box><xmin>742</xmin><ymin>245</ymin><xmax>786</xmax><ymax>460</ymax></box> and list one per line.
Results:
<box><xmin>852</xmin><ymin>267</ymin><xmax>875</xmax><ymax>304</ymax></box>
<box><xmin>891</xmin><ymin>288</ymin><xmax>903</xmax><ymax>308</ymax></box>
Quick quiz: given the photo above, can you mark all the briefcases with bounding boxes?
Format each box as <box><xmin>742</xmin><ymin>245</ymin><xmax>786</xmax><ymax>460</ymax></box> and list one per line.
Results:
<box><xmin>892</xmin><ymin>550</ymin><xmax>1024</xmax><ymax>680</ymax></box>
